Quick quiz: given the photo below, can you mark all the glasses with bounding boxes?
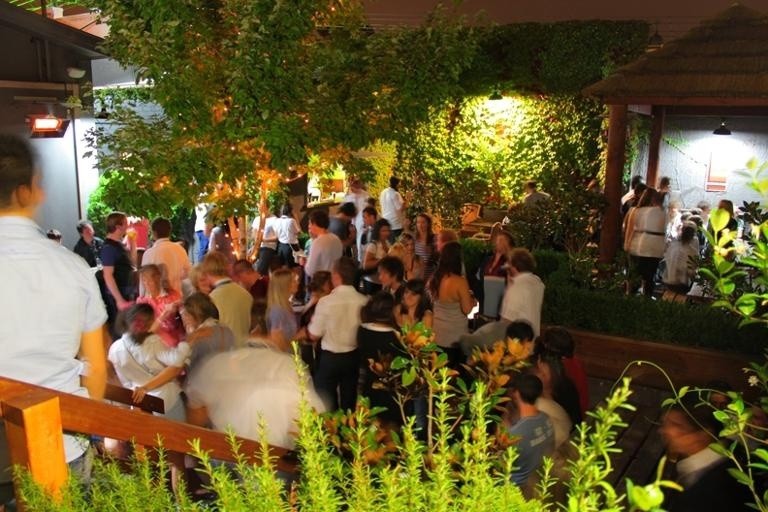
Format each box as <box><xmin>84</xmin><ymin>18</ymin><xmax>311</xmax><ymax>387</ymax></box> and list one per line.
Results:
<box><xmin>486</xmin><ymin>91</ymin><xmax>505</xmax><ymax>112</ymax></box>
<box><xmin>24</xmin><ymin>111</ymin><xmax>69</xmax><ymax>139</ymax></box>
<box><xmin>712</xmin><ymin>117</ymin><xmax>732</xmax><ymax>136</ymax></box>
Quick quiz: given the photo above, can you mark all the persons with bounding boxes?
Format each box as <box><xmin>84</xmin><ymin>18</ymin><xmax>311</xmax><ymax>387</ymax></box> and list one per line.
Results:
<box><xmin>620</xmin><ymin>176</ymin><xmax>745</xmax><ymax>300</ymax></box>
<box><xmin>657</xmin><ymin>380</ymin><xmax>761</xmax><ymax>510</ymax></box>
<box><xmin>48</xmin><ymin>176</ymin><xmax>588</xmax><ymax>510</ymax></box>
<box><xmin>0</xmin><ymin>135</ymin><xmax>109</xmax><ymax>502</ymax></box>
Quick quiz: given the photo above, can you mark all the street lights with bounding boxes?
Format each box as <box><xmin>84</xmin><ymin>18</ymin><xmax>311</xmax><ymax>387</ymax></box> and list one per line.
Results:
<box><xmin>293</xmin><ymin>248</ymin><xmax>304</xmax><ymax>263</ymax></box>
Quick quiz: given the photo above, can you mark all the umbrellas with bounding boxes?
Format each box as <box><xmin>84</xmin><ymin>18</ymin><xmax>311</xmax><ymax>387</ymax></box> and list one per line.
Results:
<box><xmin>192</xmin><ymin>489</ymin><xmax>217</xmax><ymax>501</ymax></box>
